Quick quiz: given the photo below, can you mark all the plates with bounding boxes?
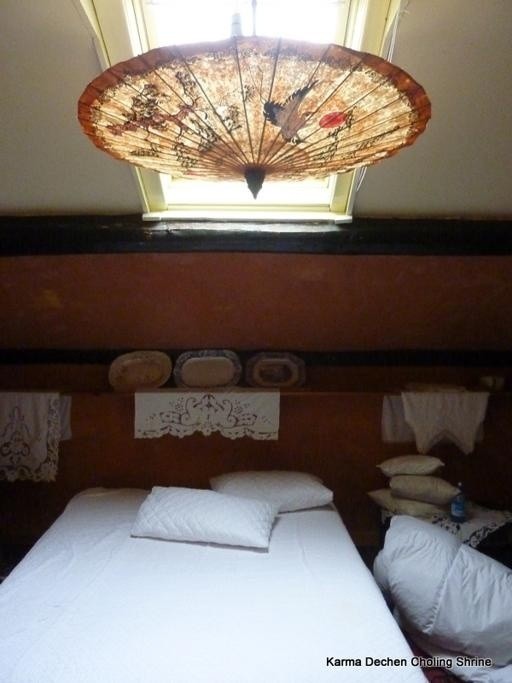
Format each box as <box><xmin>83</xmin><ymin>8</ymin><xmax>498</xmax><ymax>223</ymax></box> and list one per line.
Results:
<box><xmin>108</xmin><ymin>350</ymin><xmax>306</xmax><ymax>392</ymax></box>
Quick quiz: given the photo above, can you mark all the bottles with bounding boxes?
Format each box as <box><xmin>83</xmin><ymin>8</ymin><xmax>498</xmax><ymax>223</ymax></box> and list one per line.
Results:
<box><xmin>450</xmin><ymin>481</ymin><xmax>467</xmax><ymax>524</ymax></box>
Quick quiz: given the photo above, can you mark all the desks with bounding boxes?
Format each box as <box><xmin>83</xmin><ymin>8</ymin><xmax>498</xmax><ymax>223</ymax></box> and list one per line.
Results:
<box><xmin>379</xmin><ymin>505</ymin><xmax>512</xmax><ymax>569</ymax></box>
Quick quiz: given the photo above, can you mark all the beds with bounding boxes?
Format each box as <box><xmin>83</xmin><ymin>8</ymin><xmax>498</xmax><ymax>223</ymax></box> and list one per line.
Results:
<box><xmin>0</xmin><ymin>483</ymin><xmax>426</xmax><ymax>682</ymax></box>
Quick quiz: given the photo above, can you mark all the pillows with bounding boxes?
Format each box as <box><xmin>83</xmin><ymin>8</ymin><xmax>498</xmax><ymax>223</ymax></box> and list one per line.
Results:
<box><xmin>370</xmin><ymin>456</ymin><xmax>462</xmax><ymax>519</ymax></box>
<box><xmin>206</xmin><ymin>467</ymin><xmax>335</xmax><ymax>514</ymax></box>
<box><xmin>130</xmin><ymin>482</ymin><xmax>280</xmax><ymax>550</ymax></box>
